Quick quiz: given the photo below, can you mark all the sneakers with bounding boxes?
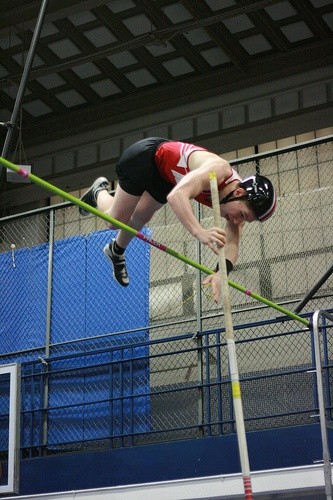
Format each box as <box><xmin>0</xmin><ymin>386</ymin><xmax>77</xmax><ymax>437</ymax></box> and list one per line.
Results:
<box><xmin>79</xmin><ymin>177</ymin><xmax>109</xmax><ymax>216</ymax></box>
<box><xmin>103</xmin><ymin>239</ymin><xmax>130</xmax><ymax>286</ymax></box>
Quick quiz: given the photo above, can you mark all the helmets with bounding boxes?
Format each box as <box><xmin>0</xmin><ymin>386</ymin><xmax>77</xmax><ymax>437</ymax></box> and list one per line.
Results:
<box><xmin>239</xmin><ymin>175</ymin><xmax>276</xmax><ymax>223</ymax></box>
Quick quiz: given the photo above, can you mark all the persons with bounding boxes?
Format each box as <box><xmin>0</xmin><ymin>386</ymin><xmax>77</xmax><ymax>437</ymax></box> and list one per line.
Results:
<box><xmin>78</xmin><ymin>135</ymin><xmax>278</xmax><ymax>305</ymax></box>
